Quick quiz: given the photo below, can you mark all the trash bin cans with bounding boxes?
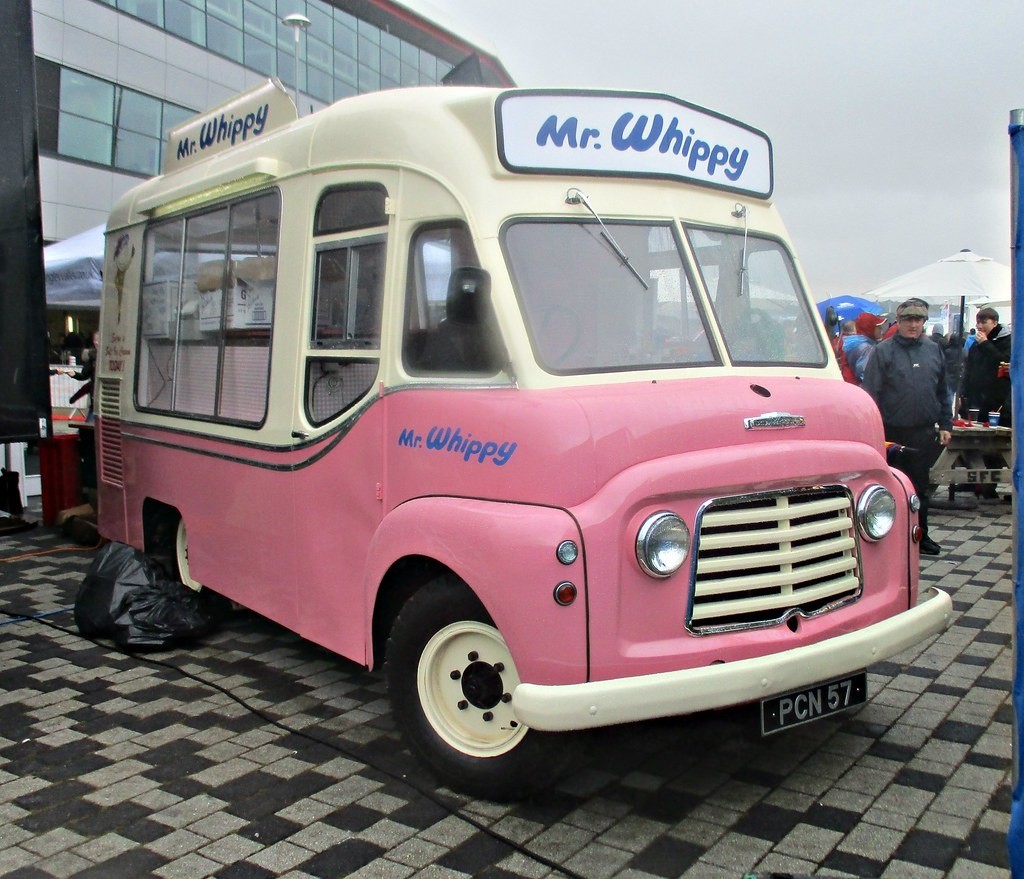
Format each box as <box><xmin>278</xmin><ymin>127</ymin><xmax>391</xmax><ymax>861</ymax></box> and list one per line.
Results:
<box><xmin>38</xmin><ymin>434</ymin><xmax>82</xmax><ymax>526</ymax></box>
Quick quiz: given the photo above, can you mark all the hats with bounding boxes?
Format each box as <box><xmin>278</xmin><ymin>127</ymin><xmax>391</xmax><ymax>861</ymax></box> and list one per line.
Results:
<box><xmin>855</xmin><ymin>313</ymin><xmax>887</xmax><ymax>337</ymax></box>
<box><xmin>932</xmin><ymin>323</ymin><xmax>944</xmax><ymax>336</ymax></box>
<box><xmin>895</xmin><ymin>298</ymin><xmax>929</xmax><ymax>321</ymax></box>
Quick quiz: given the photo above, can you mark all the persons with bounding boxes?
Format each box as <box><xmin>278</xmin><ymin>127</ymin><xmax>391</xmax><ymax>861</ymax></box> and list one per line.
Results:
<box><xmin>826</xmin><ymin>298</ymin><xmax>1012</xmax><ymax>555</ymax></box>
<box><xmin>49</xmin><ymin>331</ymin><xmax>99</xmax><ymax>422</ymax></box>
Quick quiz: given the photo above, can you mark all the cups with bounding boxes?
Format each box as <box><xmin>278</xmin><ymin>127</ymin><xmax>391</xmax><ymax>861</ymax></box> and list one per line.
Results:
<box><xmin>969</xmin><ymin>409</ymin><xmax>980</xmax><ymax>421</ymax></box>
<box><xmin>988</xmin><ymin>412</ymin><xmax>1001</xmax><ymax>426</ymax></box>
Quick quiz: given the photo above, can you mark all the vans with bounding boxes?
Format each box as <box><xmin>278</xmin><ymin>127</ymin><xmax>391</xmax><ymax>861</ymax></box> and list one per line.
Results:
<box><xmin>96</xmin><ymin>78</ymin><xmax>955</xmax><ymax>802</ymax></box>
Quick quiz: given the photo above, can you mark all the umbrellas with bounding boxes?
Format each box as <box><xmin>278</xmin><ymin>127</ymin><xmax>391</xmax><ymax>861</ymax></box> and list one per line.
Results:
<box><xmin>861</xmin><ymin>248</ymin><xmax>1011</xmax><ymax>421</ymax></box>
<box><xmin>815</xmin><ymin>295</ymin><xmax>886</xmax><ymax>330</ymax></box>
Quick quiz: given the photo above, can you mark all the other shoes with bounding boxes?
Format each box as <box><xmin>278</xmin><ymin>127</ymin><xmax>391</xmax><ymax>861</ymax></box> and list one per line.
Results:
<box><xmin>919</xmin><ymin>535</ymin><xmax>940</xmax><ymax>554</ymax></box>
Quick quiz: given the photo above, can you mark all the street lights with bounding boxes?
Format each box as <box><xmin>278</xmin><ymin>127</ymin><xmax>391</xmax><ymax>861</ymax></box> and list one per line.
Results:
<box><xmin>281</xmin><ymin>13</ymin><xmax>312</xmax><ymax>115</ymax></box>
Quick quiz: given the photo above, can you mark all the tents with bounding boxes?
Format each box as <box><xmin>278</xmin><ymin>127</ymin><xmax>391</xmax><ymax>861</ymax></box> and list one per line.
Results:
<box><xmin>657</xmin><ymin>274</ymin><xmax>797</xmax><ymax>315</ymax></box>
<box><xmin>44</xmin><ymin>222</ymin><xmax>451</xmax><ymax>311</ymax></box>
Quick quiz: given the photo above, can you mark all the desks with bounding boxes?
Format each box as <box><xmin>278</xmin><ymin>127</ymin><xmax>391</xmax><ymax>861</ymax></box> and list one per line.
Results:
<box><xmin>922</xmin><ymin>418</ymin><xmax>1013</xmax><ymax>500</ymax></box>
